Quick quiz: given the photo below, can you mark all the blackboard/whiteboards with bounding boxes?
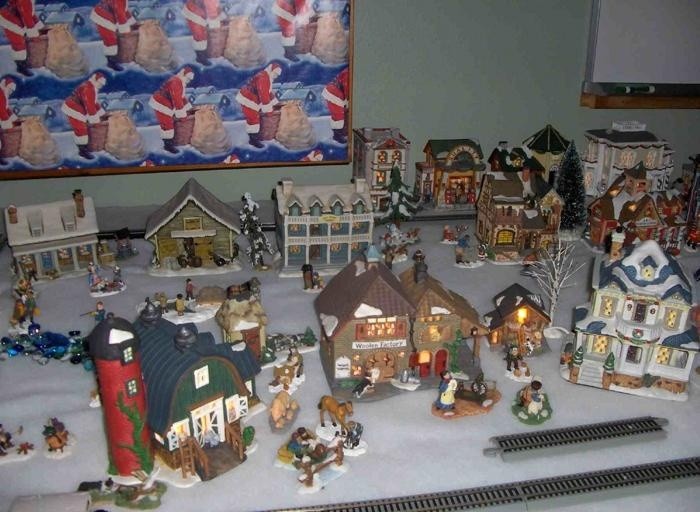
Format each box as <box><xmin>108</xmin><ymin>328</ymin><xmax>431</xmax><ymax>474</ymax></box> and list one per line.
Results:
<box><xmin>583</xmin><ymin>1</ymin><xmax>699</xmax><ymax>97</ymax></box>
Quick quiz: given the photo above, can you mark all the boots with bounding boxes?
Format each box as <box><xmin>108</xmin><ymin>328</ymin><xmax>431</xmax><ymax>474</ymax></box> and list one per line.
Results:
<box><xmin>249</xmin><ymin>133</ymin><xmax>264</xmax><ymax>148</ymax></box>
<box><xmin>15</xmin><ymin>59</ymin><xmax>34</xmax><ymax>77</ymax></box>
<box><xmin>332</xmin><ymin>130</ymin><xmax>348</xmax><ymax>144</ymax></box>
<box><xmin>78</xmin><ymin>144</ymin><xmax>96</xmax><ymax>159</ymax></box>
<box><xmin>284</xmin><ymin>46</ymin><xmax>301</xmax><ymax>62</ymax></box>
<box><xmin>106</xmin><ymin>56</ymin><xmax>125</xmax><ymax>72</ymax></box>
<box><xmin>163</xmin><ymin>139</ymin><xmax>180</xmax><ymax>154</ymax></box>
<box><xmin>196</xmin><ymin>49</ymin><xmax>212</xmax><ymax>66</ymax></box>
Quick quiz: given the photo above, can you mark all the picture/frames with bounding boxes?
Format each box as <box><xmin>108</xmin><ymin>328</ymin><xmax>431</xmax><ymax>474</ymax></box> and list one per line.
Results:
<box><xmin>0</xmin><ymin>0</ymin><xmax>359</xmax><ymax>183</ymax></box>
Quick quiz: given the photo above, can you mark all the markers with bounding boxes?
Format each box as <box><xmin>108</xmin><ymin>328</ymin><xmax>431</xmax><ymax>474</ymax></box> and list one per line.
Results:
<box><xmin>616</xmin><ymin>86</ymin><xmax>657</xmax><ymax>93</ymax></box>
